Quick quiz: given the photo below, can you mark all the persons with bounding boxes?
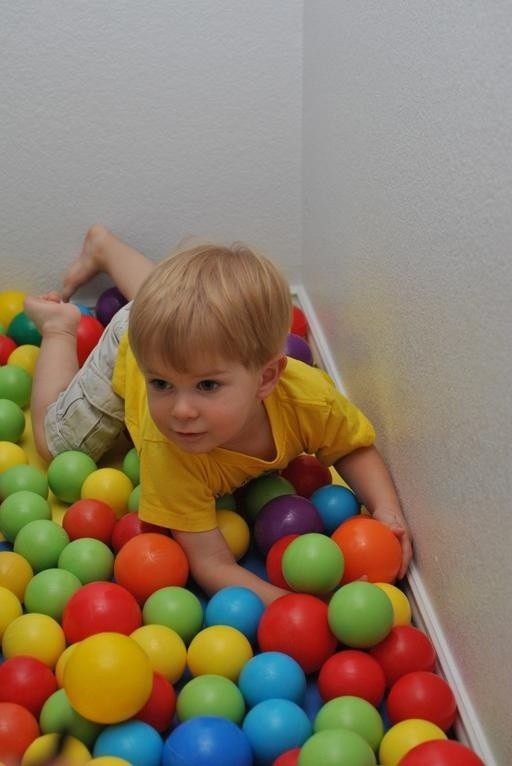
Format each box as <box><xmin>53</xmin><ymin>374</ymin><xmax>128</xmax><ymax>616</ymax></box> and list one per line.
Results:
<box><xmin>24</xmin><ymin>225</ymin><xmax>412</xmax><ymax>604</ymax></box>
